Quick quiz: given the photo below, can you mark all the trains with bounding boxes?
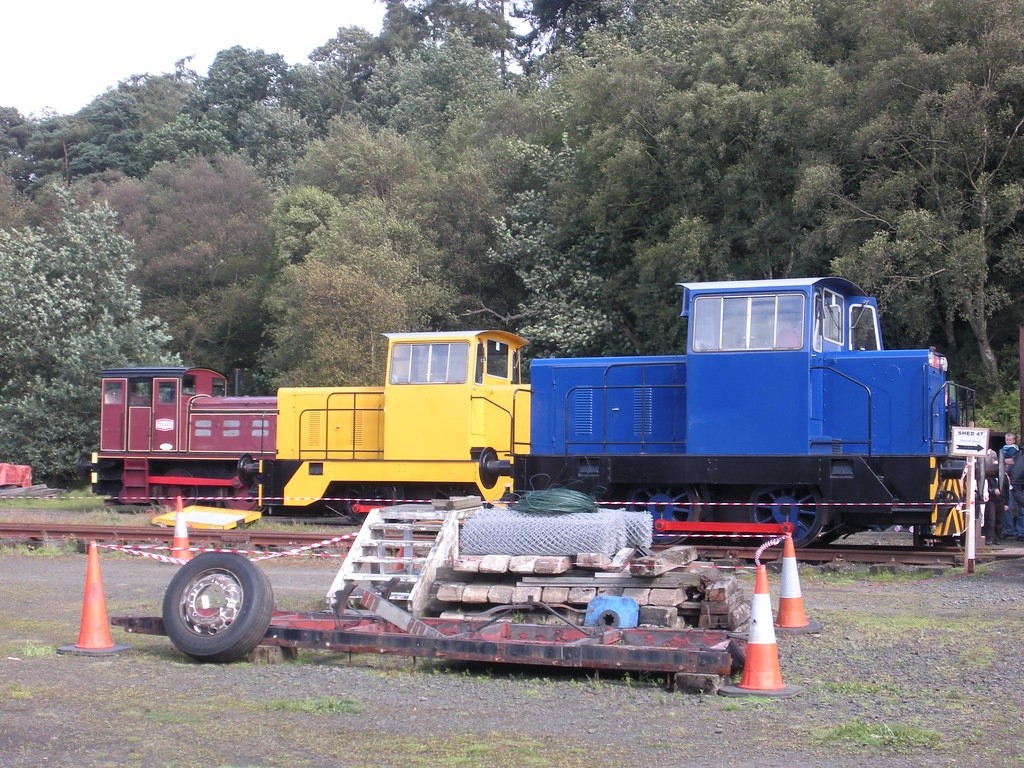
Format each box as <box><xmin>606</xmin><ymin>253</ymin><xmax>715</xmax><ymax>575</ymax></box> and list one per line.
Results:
<box><xmin>82</xmin><ymin>276</ymin><xmax>1007</xmax><ymax>551</ymax></box>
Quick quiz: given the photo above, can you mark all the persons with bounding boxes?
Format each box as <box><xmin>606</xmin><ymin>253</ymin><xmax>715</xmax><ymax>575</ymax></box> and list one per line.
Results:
<box><xmin>979</xmin><ymin>446</ymin><xmax>1023</xmax><ymax>546</ymax></box>
<box><xmin>1003</xmin><ymin>432</ymin><xmax>1020</xmax><ymax>475</ymax></box>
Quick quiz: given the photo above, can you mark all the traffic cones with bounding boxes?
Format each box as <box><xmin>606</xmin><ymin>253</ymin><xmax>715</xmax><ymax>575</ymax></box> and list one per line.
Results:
<box><xmin>717</xmin><ymin>565</ymin><xmax>805</xmax><ymax>699</ymax></box>
<box><xmin>54</xmin><ymin>540</ymin><xmax>132</xmax><ymax>658</ymax></box>
<box><xmin>771</xmin><ymin>530</ymin><xmax>824</xmax><ymax>636</ymax></box>
<box><xmin>167</xmin><ymin>497</ymin><xmax>194</xmax><ymax>562</ymax></box>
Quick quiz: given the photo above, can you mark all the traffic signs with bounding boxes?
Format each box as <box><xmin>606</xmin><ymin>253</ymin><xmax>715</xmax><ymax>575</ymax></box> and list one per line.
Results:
<box><xmin>951</xmin><ymin>425</ymin><xmax>989</xmax><ymax>459</ymax></box>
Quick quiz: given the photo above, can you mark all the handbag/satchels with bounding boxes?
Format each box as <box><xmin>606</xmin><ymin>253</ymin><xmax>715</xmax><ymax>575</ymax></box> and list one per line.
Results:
<box><xmin>1009</xmin><ymin>487</ymin><xmax>1024</xmax><ymax>506</ymax></box>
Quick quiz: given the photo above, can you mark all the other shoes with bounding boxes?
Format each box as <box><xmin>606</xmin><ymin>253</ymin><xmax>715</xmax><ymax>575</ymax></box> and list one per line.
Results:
<box><xmin>999</xmin><ymin>534</ymin><xmax>1005</xmax><ymax>539</ymax></box>
<box><xmin>1018</xmin><ymin>537</ymin><xmax>1024</xmax><ymax>541</ymax></box>
<box><xmin>992</xmin><ymin>540</ymin><xmax>1000</xmax><ymax>545</ymax></box>
<box><xmin>985</xmin><ymin>540</ymin><xmax>992</xmax><ymax>544</ymax></box>
